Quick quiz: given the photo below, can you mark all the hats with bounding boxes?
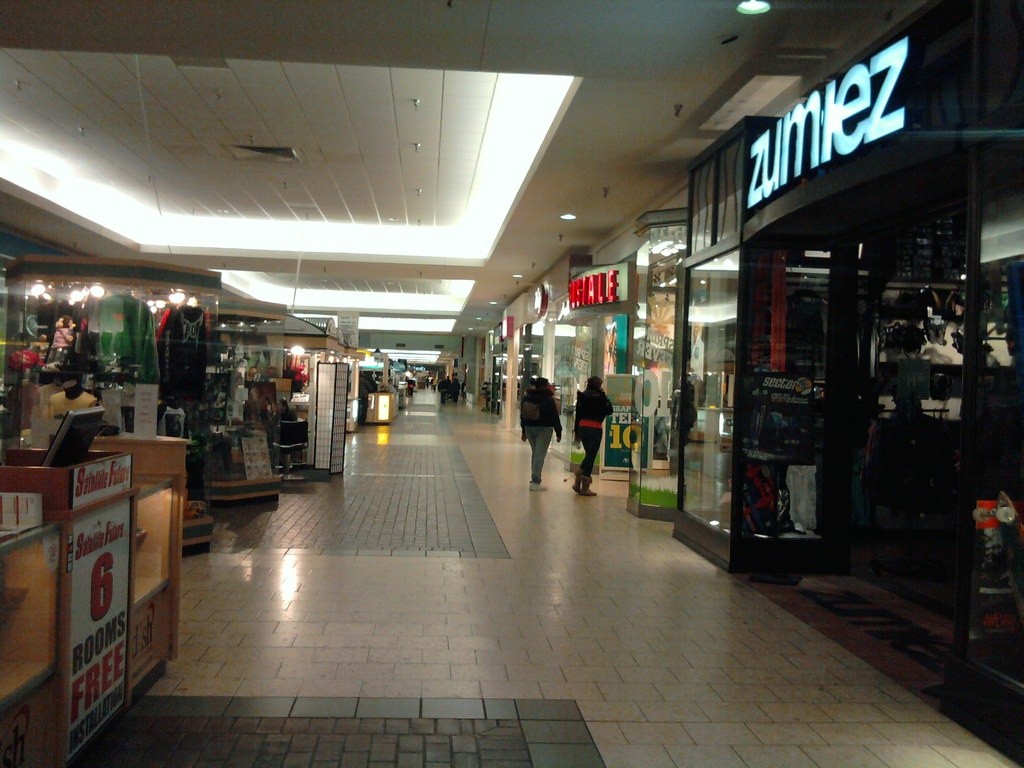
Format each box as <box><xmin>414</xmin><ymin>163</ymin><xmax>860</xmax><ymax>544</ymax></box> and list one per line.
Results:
<box><xmin>588</xmin><ymin>376</ymin><xmax>603</xmax><ymax>389</ymax></box>
<box><xmin>534</xmin><ymin>376</ymin><xmax>548</xmax><ymax>387</ymax></box>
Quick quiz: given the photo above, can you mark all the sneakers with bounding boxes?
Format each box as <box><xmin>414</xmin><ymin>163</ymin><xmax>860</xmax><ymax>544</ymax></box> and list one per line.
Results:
<box><xmin>529</xmin><ymin>482</ymin><xmax>547</xmax><ymax>491</ymax></box>
<box><xmin>930</xmin><ymin>343</ymin><xmax>963</xmax><ymax>365</ymax></box>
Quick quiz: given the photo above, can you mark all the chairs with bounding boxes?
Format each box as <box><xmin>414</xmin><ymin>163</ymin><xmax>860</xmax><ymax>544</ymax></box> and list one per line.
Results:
<box><xmin>271</xmin><ymin>420</ymin><xmax>309</xmax><ymax>470</ymax></box>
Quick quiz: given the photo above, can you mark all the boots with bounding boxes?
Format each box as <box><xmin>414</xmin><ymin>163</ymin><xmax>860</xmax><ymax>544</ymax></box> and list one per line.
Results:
<box><xmin>579</xmin><ymin>475</ymin><xmax>597</xmax><ymax>496</ymax></box>
<box><xmin>572</xmin><ymin>468</ymin><xmax>583</xmax><ymax>493</ymax></box>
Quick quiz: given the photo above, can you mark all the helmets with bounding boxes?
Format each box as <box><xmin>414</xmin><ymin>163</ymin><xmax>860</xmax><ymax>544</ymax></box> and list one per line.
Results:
<box><xmin>884</xmin><ymin>286</ymin><xmax>965</xmax><ymax>354</ymax></box>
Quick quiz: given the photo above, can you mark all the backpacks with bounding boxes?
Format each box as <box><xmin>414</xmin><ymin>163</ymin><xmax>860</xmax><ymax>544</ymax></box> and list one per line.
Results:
<box><xmin>775</xmin><ymin>487</ymin><xmax>793</xmax><ymax>534</ymax></box>
<box><xmin>521</xmin><ymin>402</ymin><xmax>541</xmax><ymax>421</ymax></box>
<box><xmin>734</xmin><ymin>458</ymin><xmax>777</xmax><ymax>540</ymax></box>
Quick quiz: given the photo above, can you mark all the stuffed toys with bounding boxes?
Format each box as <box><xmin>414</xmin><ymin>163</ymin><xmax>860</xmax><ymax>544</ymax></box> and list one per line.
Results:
<box><xmin>39</xmin><ymin>315</ymin><xmax>77</xmax><ymax>369</ymax></box>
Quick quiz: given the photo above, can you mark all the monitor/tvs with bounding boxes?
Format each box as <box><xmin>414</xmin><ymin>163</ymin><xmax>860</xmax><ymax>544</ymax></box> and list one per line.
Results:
<box><xmin>41</xmin><ymin>405</ymin><xmax>105</xmax><ymax>467</ymax></box>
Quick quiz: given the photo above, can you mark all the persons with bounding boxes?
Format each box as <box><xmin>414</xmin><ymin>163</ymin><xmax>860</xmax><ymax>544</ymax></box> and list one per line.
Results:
<box><xmin>572</xmin><ymin>376</ymin><xmax>613</xmax><ymax>496</ymax></box>
<box><xmin>358</xmin><ymin>371</ymin><xmax>377</xmax><ymax>425</ymax></box>
<box><xmin>438</xmin><ymin>376</ymin><xmax>466</xmax><ymax>404</ymax></box>
<box><xmin>37</xmin><ymin>381</ymin><xmax>98</xmax><ymax>420</ymax></box>
<box><xmin>520</xmin><ymin>377</ymin><xmax>562</xmax><ymax>491</ymax></box>
<box><xmin>268</xmin><ymin>397</ymin><xmax>297</xmax><ymax>471</ymax></box>
